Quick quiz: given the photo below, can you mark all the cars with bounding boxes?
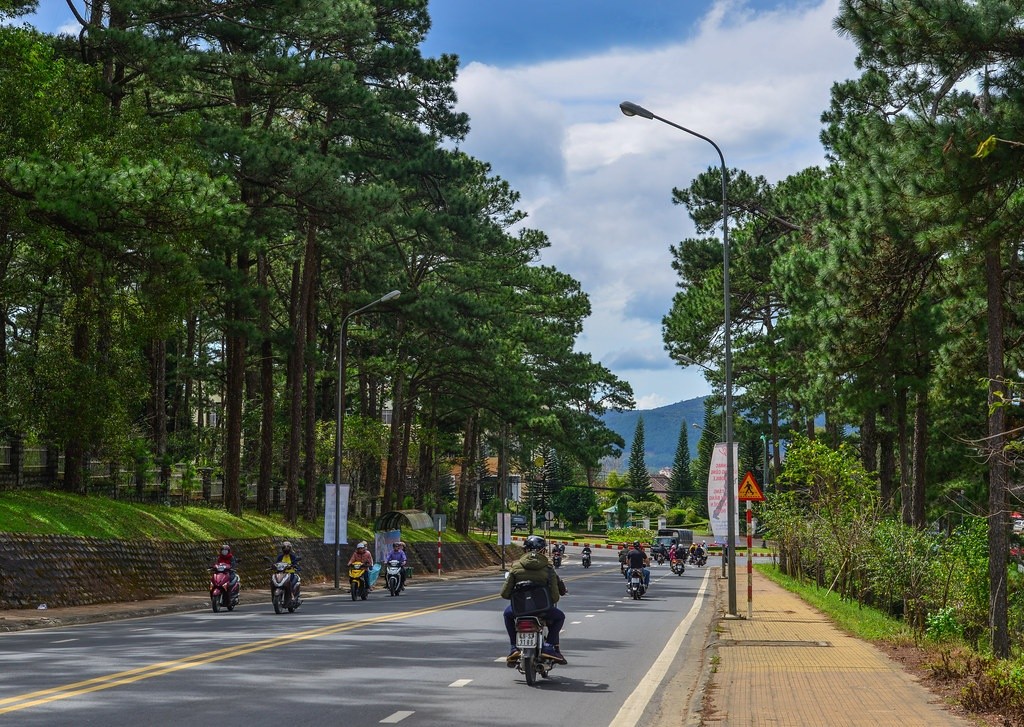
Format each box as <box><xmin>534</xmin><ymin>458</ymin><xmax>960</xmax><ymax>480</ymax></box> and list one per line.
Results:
<box><xmin>1009</xmin><ymin>511</ymin><xmax>1024</xmax><ymax>566</ymax></box>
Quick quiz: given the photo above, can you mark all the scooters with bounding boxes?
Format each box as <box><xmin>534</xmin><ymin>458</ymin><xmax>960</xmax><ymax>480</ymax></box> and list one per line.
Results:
<box><xmin>264</xmin><ymin>556</ymin><xmax>304</xmax><ymax>614</ymax></box>
<box><xmin>581</xmin><ymin>550</ymin><xmax>592</xmax><ymax>568</ymax></box>
<box><xmin>383</xmin><ymin>558</ymin><xmax>407</xmax><ymax>595</ymax></box>
<box><xmin>205</xmin><ymin>555</ymin><xmax>243</xmax><ymax>613</ymax></box>
<box><xmin>618</xmin><ymin>546</ymin><xmax>709</xmax><ymax>582</ymax></box>
<box><xmin>624</xmin><ymin>555</ymin><xmax>654</xmax><ymax>598</ymax></box>
<box><xmin>551</xmin><ymin>549</ymin><xmax>563</xmax><ymax>569</ymax></box>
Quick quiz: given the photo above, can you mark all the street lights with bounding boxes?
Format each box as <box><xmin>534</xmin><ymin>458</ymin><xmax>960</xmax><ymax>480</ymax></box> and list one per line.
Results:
<box><xmin>677</xmin><ymin>353</ymin><xmax>726</xmax><ymax>577</ymax></box>
<box><xmin>618</xmin><ymin>101</ymin><xmax>738</xmax><ymax>615</ymax></box>
<box><xmin>335</xmin><ymin>290</ymin><xmax>402</xmax><ymax>590</ymax></box>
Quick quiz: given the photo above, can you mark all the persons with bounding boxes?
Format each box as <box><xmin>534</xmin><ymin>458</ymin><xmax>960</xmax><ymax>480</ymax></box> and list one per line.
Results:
<box><xmin>659</xmin><ymin>541</ymin><xmax>668</xmax><ymax>556</ymax></box>
<box><xmin>618</xmin><ymin>541</ymin><xmax>630</xmax><ymax>574</ymax></box>
<box><xmin>626</xmin><ymin>539</ymin><xmax>650</xmax><ymax>591</ymax></box>
<box><xmin>560</xmin><ymin>544</ymin><xmax>565</xmax><ymax>553</ymax></box>
<box><xmin>347</xmin><ymin>540</ymin><xmax>373</xmax><ymax>589</ymax></box>
<box><xmin>500</xmin><ymin>534</ymin><xmax>567</xmax><ymax>664</ymax></box>
<box><xmin>217</xmin><ymin>545</ymin><xmax>235</xmax><ymax>582</ymax></box>
<box><xmin>670</xmin><ymin>538</ymin><xmax>709</xmax><ymax>571</ymax></box>
<box><xmin>383</xmin><ymin>541</ymin><xmax>407</xmax><ymax>591</ymax></box>
<box><xmin>581</xmin><ymin>544</ymin><xmax>591</xmax><ymax>565</ymax></box>
<box><xmin>552</xmin><ymin>545</ymin><xmax>562</xmax><ymax>565</ymax></box>
<box><xmin>271</xmin><ymin>541</ymin><xmax>302</xmax><ymax>601</ymax></box>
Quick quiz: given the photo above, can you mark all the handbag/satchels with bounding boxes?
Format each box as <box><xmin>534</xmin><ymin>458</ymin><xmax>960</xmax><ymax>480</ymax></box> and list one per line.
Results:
<box><xmin>510</xmin><ymin>585</ymin><xmax>550</xmax><ymax>617</ymax></box>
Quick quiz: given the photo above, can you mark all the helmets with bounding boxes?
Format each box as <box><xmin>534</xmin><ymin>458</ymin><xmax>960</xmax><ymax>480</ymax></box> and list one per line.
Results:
<box><xmin>393</xmin><ymin>542</ymin><xmax>399</xmax><ymax>548</ymax></box>
<box><xmin>678</xmin><ymin>544</ymin><xmax>683</xmax><ymax>549</ymax></box>
<box><xmin>633</xmin><ymin>540</ymin><xmax>640</xmax><ymax>547</ymax></box>
<box><xmin>623</xmin><ymin>542</ymin><xmax>629</xmax><ymax>548</ymax></box>
<box><xmin>219</xmin><ymin>545</ymin><xmax>230</xmax><ymax>555</ymax></box>
<box><xmin>399</xmin><ymin>542</ymin><xmax>405</xmax><ymax>547</ymax></box>
<box><xmin>362</xmin><ymin>541</ymin><xmax>367</xmax><ymax>549</ymax></box>
<box><xmin>357</xmin><ymin>543</ymin><xmax>364</xmax><ymax>549</ymax></box>
<box><xmin>282</xmin><ymin>542</ymin><xmax>291</xmax><ymax>548</ymax></box>
<box><xmin>524</xmin><ymin>536</ymin><xmax>545</xmax><ymax>555</ymax></box>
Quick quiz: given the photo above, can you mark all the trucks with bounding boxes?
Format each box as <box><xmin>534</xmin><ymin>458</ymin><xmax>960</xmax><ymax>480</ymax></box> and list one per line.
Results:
<box><xmin>652</xmin><ymin>528</ymin><xmax>693</xmax><ymax>550</ymax></box>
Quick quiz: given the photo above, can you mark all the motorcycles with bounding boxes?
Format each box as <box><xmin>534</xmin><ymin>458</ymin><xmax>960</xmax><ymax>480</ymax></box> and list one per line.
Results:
<box><xmin>503</xmin><ymin>570</ymin><xmax>568</xmax><ymax>687</ymax></box>
<box><xmin>345</xmin><ymin>561</ymin><xmax>371</xmax><ymax>602</ymax></box>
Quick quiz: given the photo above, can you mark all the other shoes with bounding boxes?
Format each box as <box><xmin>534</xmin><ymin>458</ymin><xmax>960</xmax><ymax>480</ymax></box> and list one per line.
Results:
<box><xmin>367</xmin><ymin>588</ymin><xmax>371</xmax><ymax>593</ymax></box>
<box><xmin>347</xmin><ymin>590</ymin><xmax>352</xmax><ymax>593</ymax></box>
<box><xmin>507</xmin><ymin>645</ymin><xmax>520</xmax><ymax>662</ymax></box>
<box><xmin>400</xmin><ymin>585</ymin><xmax>405</xmax><ymax>591</ymax></box>
<box><xmin>291</xmin><ymin>593</ymin><xmax>295</xmax><ymax>601</ymax></box>
<box><xmin>383</xmin><ymin>586</ymin><xmax>388</xmax><ymax>588</ymax></box>
<box><xmin>542</xmin><ymin>642</ymin><xmax>563</xmax><ymax>661</ymax></box>
<box><xmin>507</xmin><ymin>662</ymin><xmax>516</xmax><ymax>667</ymax></box>
<box><xmin>554</xmin><ymin>646</ymin><xmax>567</xmax><ymax>665</ymax></box>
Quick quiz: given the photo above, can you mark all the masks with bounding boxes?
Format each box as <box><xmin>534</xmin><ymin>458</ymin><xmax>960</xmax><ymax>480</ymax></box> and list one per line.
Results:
<box><xmin>221</xmin><ymin>550</ymin><xmax>228</xmax><ymax>555</ymax></box>
